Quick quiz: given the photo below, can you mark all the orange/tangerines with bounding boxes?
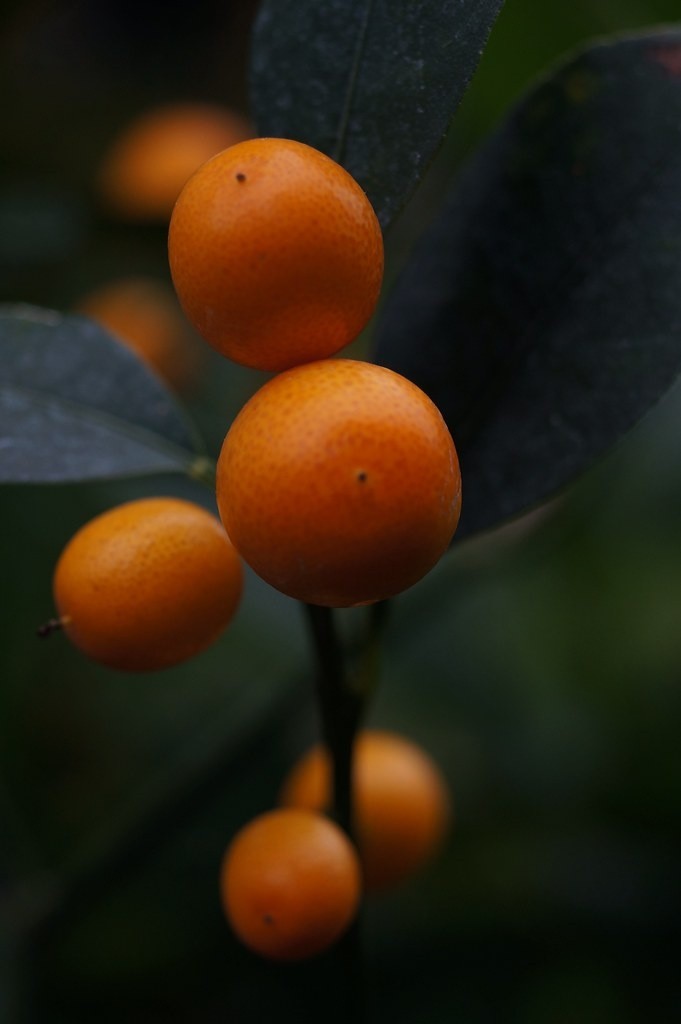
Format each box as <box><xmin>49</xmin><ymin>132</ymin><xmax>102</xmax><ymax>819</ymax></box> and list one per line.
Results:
<box><xmin>55</xmin><ymin>116</ymin><xmax>463</xmax><ymax>953</ymax></box>
